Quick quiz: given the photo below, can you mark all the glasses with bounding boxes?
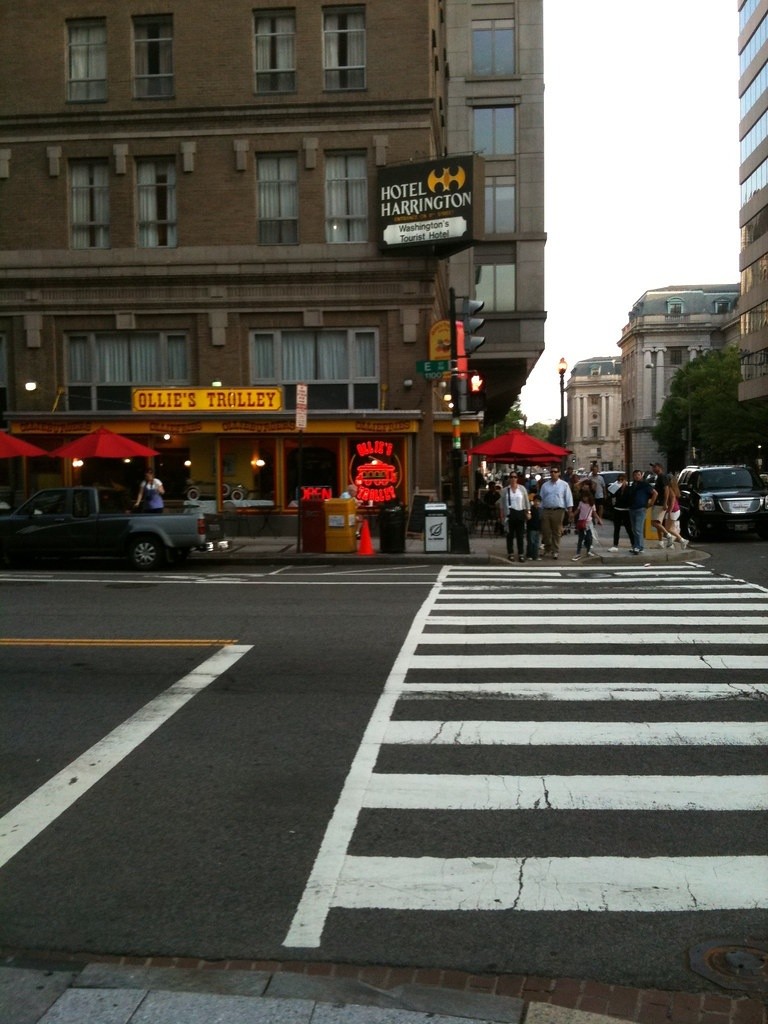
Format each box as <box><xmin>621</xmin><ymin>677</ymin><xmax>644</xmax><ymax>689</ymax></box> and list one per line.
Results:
<box><xmin>617</xmin><ymin>479</ymin><xmax>624</xmax><ymax>483</ymax></box>
<box><xmin>510</xmin><ymin>476</ymin><xmax>517</xmax><ymax>479</ymax></box>
<box><xmin>550</xmin><ymin>471</ymin><xmax>558</xmax><ymax>474</ymax></box>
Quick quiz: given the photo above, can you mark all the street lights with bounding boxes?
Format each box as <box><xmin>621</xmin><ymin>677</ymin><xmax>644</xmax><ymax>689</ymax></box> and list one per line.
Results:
<box><xmin>557</xmin><ymin>358</ymin><xmax>567</xmax><ymax>447</ymax></box>
<box><xmin>522</xmin><ymin>414</ymin><xmax>527</xmax><ymax>433</ymax></box>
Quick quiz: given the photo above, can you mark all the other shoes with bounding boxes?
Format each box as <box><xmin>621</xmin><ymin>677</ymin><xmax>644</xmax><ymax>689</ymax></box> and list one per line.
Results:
<box><xmin>650</xmin><ymin>543</ymin><xmax>664</xmax><ymax>549</ymax></box>
<box><xmin>518</xmin><ymin>555</ymin><xmax>525</xmax><ymax>562</ymax></box>
<box><xmin>572</xmin><ymin>555</ymin><xmax>581</xmax><ymax>561</ymax></box>
<box><xmin>633</xmin><ymin>547</ymin><xmax>640</xmax><ymax>554</ymax></box>
<box><xmin>527</xmin><ymin>556</ymin><xmax>533</xmax><ymax>561</ymax></box>
<box><xmin>680</xmin><ymin>540</ymin><xmax>689</xmax><ymax>551</ymax></box>
<box><xmin>536</xmin><ymin>557</ymin><xmax>543</xmax><ymax>560</ymax></box>
<box><xmin>552</xmin><ymin>553</ymin><xmax>560</xmax><ymax>559</ymax></box>
<box><xmin>587</xmin><ymin>551</ymin><xmax>594</xmax><ymax>556</ymax></box>
<box><xmin>607</xmin><ymin>546</ymin><xmax>618</xmax><ymax>552</ymax></box>
<box><xmin>539</xmin><ymin>551</ymin><xmax>551</xmax><ymax>556</ymax></box>
<box><xmin>508</xmin><ymin>555</ymin><xmax>516</xmax><ymax>562</ymax></box>
<box><xmin>666</xmin><ymin>535</ymin><xmax>676</xmax><ymax>547</ymax></box>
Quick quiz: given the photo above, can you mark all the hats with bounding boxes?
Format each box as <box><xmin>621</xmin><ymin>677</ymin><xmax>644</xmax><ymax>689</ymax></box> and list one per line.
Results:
<box><xmin>648</xmin><ymin>462</ymin><xmax>665</xmax><ymax>469</ymax></box>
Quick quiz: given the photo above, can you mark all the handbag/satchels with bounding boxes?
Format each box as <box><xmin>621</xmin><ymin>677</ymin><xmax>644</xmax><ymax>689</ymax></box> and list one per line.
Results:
<box><xmin>576</xmin><ymin>519</ymin><xmax>587</xmax><ymax>529</ymax></box>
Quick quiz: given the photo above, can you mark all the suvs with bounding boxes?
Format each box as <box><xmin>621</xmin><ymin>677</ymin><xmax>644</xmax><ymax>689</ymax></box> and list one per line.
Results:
<box><xmin>676</xmin><ymin>465</ymin><xmax>768</xmax><ymax>542</ymax></box>
<box><xmin>597</xmin><ymin>470</ymin><xmax>626</xmax><ymax>485</ymax></box>
<box><xmin>645</xmin><ymin>363</ymin><xmax>692</xmax><ymax>466</ymax></box>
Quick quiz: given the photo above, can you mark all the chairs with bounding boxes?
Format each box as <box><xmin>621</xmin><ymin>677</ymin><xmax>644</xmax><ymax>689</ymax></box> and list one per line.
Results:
<box><xmin>223</xmin><ymin>501</ymin><xmax>252</xmax><ymax>537</ymax></box>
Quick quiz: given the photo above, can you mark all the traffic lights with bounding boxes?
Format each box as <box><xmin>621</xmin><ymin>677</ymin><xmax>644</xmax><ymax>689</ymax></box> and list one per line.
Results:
<box><xmin>462</xmin><ymin>296</ymin><xmax>485</xmax><ymax>359</ymax></box>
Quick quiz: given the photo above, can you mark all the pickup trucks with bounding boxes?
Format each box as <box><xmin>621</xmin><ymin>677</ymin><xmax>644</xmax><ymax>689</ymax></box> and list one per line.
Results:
<box><xmin>0</xmin><ymin>486</ymin><xmax>205</xmax><ymax>572</ymax></box>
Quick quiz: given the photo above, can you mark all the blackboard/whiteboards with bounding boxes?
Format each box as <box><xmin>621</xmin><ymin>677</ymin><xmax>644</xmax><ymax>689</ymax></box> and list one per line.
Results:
<box><xmin>405</xmin><ymin>494</ymin><xmax>435</xmax><ymax>536</ymax></box>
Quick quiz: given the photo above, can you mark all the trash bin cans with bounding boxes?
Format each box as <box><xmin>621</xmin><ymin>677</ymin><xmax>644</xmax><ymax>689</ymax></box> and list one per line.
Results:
<box><xmin>424</xmin><ymin>502</ymin><xmax>447</xmax><ymax>553</ymax></box>
<box><xmin>376</xmin><ymin>506</ymin><xmax>406</xmax><ymax>552</ymax></box>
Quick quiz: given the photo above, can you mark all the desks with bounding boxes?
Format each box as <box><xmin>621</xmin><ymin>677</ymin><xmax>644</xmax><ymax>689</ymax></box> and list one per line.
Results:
<box><xmin>250</xmin><ymin>505</ymin><xmax>281</xmax><ymax>538</ymax></box>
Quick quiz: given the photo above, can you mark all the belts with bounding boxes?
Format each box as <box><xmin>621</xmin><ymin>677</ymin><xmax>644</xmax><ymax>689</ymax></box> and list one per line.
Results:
<box><xmin>543</xmin><ymin>507</ymin><xmax>566</xmax><ymax>510</ymax></box>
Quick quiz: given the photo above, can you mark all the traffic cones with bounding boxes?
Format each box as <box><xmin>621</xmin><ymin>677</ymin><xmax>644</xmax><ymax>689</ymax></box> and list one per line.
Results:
<box><xmin>357</xmin><ymin>520</ymin><xmax>374</xmax><ymax>555</ymax></box>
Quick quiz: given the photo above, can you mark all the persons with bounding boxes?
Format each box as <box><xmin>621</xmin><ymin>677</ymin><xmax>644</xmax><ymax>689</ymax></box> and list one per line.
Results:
<box><xmin>649</xmin><ymin>462</ymin><xmax>677</xmax><ymax>549</ymax></box>
<box><xmin>540</xmin><ymin>466</ymin><xmax>573</xmax><ymax>558</ymax></box>
<box><xmin>571</xmin><ymin>490</ymin><xmax>604</xmax><ymax>556</ymax></box>
<box><xmin>485</xmin><ymin>480</ymin><xmax>501</xmax><ymax>504</ymax></box>
<box><xmin>662</xmin><ymin>473</ymin><xmax>690</xmax><ymax>550</ymax></box>
<box><xmin>630</xmin><ymin>469</ymin><xmax>658</xmax><ymax>552</ymax></box>
<box><xmin>607</xmin><ymin>474</ymin><xmax>633</xmax><ymax>552</ymax></box>
<box><xmin>525</xmin><ymin>494</ymin><xmax>543</xmax><ymax>561</ymax></box>
<box><xmin>588</xmin><ymin>466</ymin><xmax>606</xmax><ymax>526</ymax></box>
<box><xmin>499</xmin><ymin>470</ymin><xmax>531</xmax><ymax>562</ymax></box>
<box><xmin>133</xmin><ymin>466</ymin><xmax>166</xmax><ymax>513</ymax></box>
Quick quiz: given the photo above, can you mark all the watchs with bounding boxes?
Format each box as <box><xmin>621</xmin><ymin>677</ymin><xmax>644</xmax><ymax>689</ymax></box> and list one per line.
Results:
<box><xmin>526</xmin><ymin>511</ymin><xmax>531</xmax><ymax>513</ymax></box>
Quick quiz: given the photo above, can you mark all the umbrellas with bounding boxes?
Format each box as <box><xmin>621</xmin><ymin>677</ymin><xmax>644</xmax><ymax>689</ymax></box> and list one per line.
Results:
<box><xmin>465</xmin><ymin>428</ymin><xmax>574</xmax><ymax>466</ymax></box>
<box><xmin>0</xmin><ymin>430</ymin><xmax>49</xmax><ymax>458</ymax></box>
<box><xmin>50</xmin><ymin>425</ymin><xmax>161</xmax><ymax>459</ymax></box>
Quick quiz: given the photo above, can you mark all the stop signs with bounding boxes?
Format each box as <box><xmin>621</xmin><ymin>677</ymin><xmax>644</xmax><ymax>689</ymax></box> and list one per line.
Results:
<box><xmin>471</xmin><ymin>375</ymin><xmax>483</xmax><ymax>391</ymax></box>
